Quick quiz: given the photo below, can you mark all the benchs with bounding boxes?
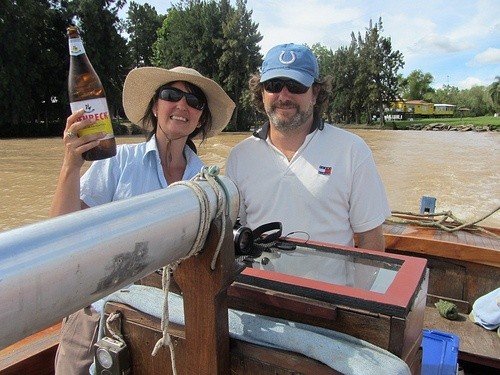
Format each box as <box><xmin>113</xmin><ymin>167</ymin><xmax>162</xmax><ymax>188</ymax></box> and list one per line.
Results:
<box><xmin>424</xmin><ymin>306</ymin><xmax>500</xmax><ymax>368</ymax></box>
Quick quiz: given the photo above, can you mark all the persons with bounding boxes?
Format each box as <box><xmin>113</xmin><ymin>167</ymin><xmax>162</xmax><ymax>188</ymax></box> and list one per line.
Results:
<box><xmin>225</xmin><ymin>43</ymin><xmax>390</xmax><ymax>291</ymax></box>
<box><xmin>48</xmin><ymin>66</ymin><xmax>236</xmax><ymax>375</ymax></box>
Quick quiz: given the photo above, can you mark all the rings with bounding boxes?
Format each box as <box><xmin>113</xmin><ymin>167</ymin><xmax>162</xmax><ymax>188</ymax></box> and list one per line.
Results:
<box><xmin>68</xmin><ymin>129</ymin><xmax>75</xmax><ymax>137</ymax></box>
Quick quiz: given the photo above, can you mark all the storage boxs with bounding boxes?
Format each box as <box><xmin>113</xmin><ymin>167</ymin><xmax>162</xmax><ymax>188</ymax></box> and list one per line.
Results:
<box><xmin>420</xmin><ymin>328</ymin><xmax>460</xmax><ymax>375</ymax></box>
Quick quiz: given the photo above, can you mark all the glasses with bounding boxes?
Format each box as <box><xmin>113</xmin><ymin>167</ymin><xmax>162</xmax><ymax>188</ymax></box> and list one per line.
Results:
<box><xmin>264</xmin><ymin>78</ymin><xmax>310</xmax><ymax>94</ymax></box>
<box><xmin>159</xmin><ymin>87</ymin><xmax>205</xmax><ymax>110</ymax></box>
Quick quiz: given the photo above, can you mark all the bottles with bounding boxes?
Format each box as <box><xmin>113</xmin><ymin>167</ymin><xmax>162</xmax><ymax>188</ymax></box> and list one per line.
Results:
<box><xmin>65</xmin><ymin>25</ymin><xmax>118</xmax><ymax>161</ymax></box>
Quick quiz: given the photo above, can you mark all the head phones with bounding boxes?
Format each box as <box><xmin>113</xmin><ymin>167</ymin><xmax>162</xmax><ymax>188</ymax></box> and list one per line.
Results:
<box><xmin>234</xmin><ymin>222</ymin><xmax>282</xmax><ymax>256</ymax></box>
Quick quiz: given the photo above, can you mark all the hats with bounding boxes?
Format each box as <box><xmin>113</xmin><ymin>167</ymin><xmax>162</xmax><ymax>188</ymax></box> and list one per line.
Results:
<box><xmin>259</xmin><ymin>42</ymin><xmax>318</xmax><ymax>87</ymax></box>
<box><xmin>122</xmin><ymin>66</ymin><xmax>235</xmax><ymax>140</ymax></box>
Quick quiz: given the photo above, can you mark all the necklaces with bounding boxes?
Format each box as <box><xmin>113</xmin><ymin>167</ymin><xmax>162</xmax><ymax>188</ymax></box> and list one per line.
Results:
<box><xmin>163</xmin><ymin>162</ymin><xmax>183</xmax><ymax>170</ymax></box>
